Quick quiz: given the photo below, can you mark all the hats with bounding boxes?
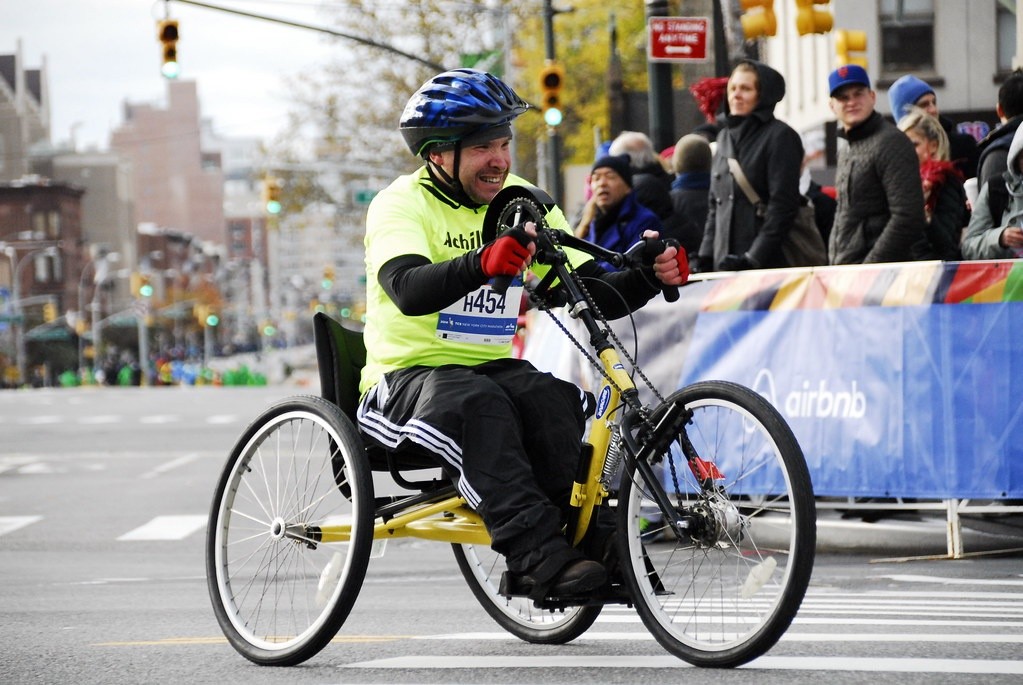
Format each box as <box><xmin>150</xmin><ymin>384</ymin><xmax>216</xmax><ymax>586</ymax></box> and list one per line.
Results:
<box><xmin>589</xmin><ymin>154</ymin><xmax>635</xmax><ymax>187</ymax></box>
<box><xmin>672</xmin><ymin>135</ymin><xmax>712</xmax><ymax>174</ymax></box>
<box><xmin>828</xmin><ymin>65</ymin><xmax>870</xmax><ymax>97</ymax></box>
<box><xmin>887</xmin><ymin>73</ymin><xmax>936</xmax><ymax>124</ymax></box>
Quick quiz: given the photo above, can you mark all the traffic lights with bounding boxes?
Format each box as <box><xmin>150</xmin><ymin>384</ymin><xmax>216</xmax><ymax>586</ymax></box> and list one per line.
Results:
<box><xmin>740</xmin><ymin>0</ymin><xmax>777</xmax><ymax>40</ymax></box>
<box><xmin>539</xmin><ymin>63</ymin><xmax>565</xmax><ymax>128</ymax></box>
<box><xmin>158</xmin><ymin>19</ymin><xmax>181</xmax><ymax>81</ymax></box>
<box><xmin>836</xmin><ymin>28</ymin><xmax>867</xmax><ymax>69</ymax></box>
<box><xmin>795</xmin><ymin>0</ymin><xmax>833</xmax><ymax>37</ymax></box>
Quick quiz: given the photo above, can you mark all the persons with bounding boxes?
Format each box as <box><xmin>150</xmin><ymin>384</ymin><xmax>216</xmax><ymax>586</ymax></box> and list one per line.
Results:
<box><xmin>685</xmin><ymin>60</ymin><xmax>806</xmax><ymax>515</ymax></box>
<box><xmin>570</xmin><ymin>112</ymin><xmax>730</xmax><ymax>543</ymax></box>
<box><xmin>793</xmin><ymin>151</ymin><xmax>838</xmax><ymax>268</ymax></box>
<box><xmin>355</xmin><ymin>67</ymin><xmax>687</xmax><ymax>604</ymax></box>
<box><xmin>827</xmin><ymin>64</ymin><xmax>927</xmax><ymax>521</ymax></box>
<box><xmin>888</xmin><ymin>67</ymin><xmax>1023</xmax><ymax>519</ymax></box>
<box><xmin>0</xmin><ymin>347</ymin><xmax>269</xmax><ymax>389</ymax></box>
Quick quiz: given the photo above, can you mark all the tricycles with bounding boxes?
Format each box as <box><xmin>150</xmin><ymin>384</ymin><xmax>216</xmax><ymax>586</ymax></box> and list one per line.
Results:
<box><xmin>204</xmin><ymin>184</ymin><xmax>818</xmax><ymax>672</ymax></box>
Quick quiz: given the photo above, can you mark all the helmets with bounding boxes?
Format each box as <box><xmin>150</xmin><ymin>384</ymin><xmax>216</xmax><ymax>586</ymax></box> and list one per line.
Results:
<box><xmin>400</xmin><ymin>68</ymin><xmax>529</xmax><ymax>155</ymax></box>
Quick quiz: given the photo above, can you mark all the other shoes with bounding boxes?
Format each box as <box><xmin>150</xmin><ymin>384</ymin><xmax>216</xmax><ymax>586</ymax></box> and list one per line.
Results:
<box><xmin>639</xmin><ymin>519</ymin><xmax>666</xmax><ymax>544</ymax></box>
<box><xmin>549</xmin><ymin>560</ymin><xmax>608</xmax><ymax>597</ymax></box>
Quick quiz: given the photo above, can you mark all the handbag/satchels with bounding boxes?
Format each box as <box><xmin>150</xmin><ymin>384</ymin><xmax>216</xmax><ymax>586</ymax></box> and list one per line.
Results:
<box><xmin>744</xmin><ymin>200</ymin><xmax>827</xmax><ymax>266</ymax></box>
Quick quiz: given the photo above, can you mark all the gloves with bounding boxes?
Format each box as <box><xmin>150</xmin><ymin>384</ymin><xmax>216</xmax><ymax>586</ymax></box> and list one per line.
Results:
<box><xmin>718</xmin><ymin>254</ymin><xmax>747</xmax><ymax>272</ymax></box>
<box><xmin>692</xmin><ymin>265</ymin><xmax>704</xmax><ymax>272</ymax></box>
